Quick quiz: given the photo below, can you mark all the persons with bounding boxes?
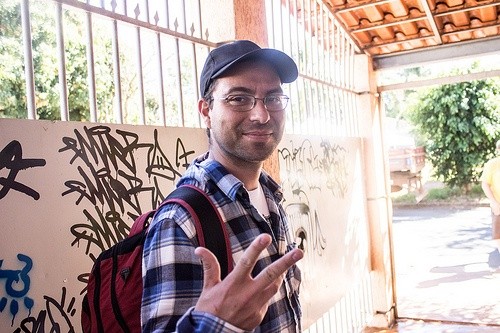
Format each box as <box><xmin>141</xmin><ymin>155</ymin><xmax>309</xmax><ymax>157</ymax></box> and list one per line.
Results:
<box><xmin>139</xmin><ymin>40</ymin><xmax>304</xmax><ymax>333</ymax></box>
<box><xmin>481</xmin><ymin>140</ymin><xmax>500</xmax><ymax>273</ymax></box>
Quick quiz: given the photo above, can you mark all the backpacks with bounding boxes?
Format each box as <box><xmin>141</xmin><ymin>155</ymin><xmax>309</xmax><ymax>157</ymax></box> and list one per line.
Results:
<box><xmin>81</xmin><ymin>184</ymin><xmax>234</xmax><ymax>333</ymax></box>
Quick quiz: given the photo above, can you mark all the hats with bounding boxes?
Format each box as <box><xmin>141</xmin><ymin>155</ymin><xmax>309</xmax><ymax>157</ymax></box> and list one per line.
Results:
<box><xmin>199</xmin><ymin>40</ymin><xmax>299</xmax><ymax>98</ymax></box>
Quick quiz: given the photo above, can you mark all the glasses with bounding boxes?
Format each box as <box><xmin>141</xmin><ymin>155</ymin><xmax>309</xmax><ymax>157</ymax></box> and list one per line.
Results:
<box><xmin>208</xmin><ymin>95</ymin><xmax>289</xmax><ymax>113</ymax></box>
